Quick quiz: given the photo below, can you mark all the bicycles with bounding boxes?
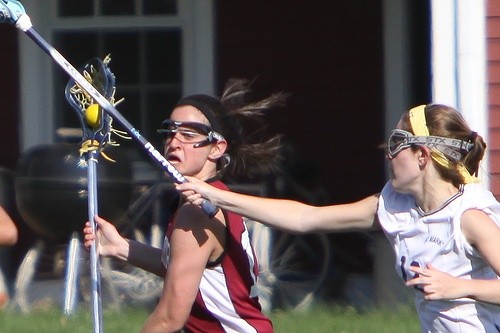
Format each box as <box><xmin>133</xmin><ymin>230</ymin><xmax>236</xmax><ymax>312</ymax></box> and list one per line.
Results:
<box><xmin>116</xmin><ymin>174</ymin><xmax>333</xmax><ymax>316</ymax></box>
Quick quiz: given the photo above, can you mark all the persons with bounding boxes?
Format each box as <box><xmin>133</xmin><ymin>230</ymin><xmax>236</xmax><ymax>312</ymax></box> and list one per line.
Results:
<box><xmin>82</xmin><ymin>73</ymin><xmax>293</xmax><ymax>333</ymax></box>
<box><xmin>0</xmin><ymin>202</ymin><xmax>19</xmax><ymax>314</ymax></box>
<box><xmin>172</xmin><ymin>103</ymin><xmax>500</xmax><ymax>332</ymax></box>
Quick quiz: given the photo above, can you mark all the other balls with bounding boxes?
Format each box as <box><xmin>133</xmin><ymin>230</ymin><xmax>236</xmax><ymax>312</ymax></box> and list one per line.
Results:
<box><xmin>84</xmin><ymin>103</ymin><xmax>102</xmax><ymax>128</ymax></box>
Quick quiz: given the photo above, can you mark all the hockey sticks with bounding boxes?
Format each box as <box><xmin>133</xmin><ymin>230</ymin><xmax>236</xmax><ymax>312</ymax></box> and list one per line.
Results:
<box><xmin>0</xmin><ymin>0</ymin><xmax>222</xmax><ymax>222</ymax></box>
<box><xmin>63</xmin><ymin>52</ymin><xmax>134</xmax><ymax>332</ymax></box>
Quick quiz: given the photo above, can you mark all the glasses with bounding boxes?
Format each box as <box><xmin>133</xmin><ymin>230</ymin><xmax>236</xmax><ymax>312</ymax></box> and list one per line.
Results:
<box><xmin>387</xmin><ymin>129</ymin><xmax>473</xmax><ymax>159</ymax></box>
<box><xmin>156</xmin><ymin>119</ymin><xmax>211</xmax><ymax>143</ymax></box>
